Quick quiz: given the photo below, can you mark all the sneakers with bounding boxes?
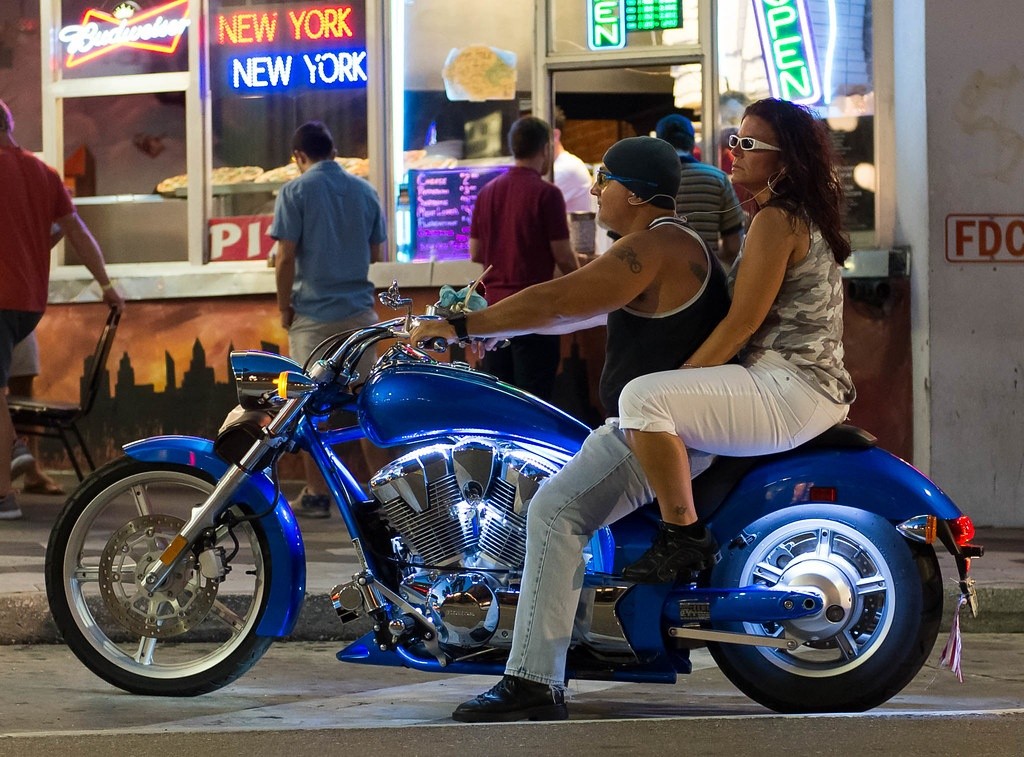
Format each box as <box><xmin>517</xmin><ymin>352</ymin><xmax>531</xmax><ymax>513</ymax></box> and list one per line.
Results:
<box><xmin>623</xmin><ymin>518</ymin><xmax>714</xmax><ymax>585</ymax></box>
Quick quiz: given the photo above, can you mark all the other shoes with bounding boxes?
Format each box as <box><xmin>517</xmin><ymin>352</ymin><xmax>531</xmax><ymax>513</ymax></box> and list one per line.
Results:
<box><xmin>9</xmin><ymin>439</ymin><xmax>36</xmax><ymax>481</ymax></box>
<box><xmin>287</xmin><ymin>485</ymin><xmax>332</xmax><ymax>519</ymax></box>
<box><xmin>0</xmin><ymin>494</ymin><xmax>23</xmax><ymax>519</ymax></box>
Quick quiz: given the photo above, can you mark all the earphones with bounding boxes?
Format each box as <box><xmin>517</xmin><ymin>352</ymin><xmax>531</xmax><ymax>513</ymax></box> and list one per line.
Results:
<box><xmin>781</xmin><ymin>166</ymin><xmax>788</xmax><ymax>174</ymax></box>
<box><xmin>632</xmin><ymin>192</ymin><xmax>638</xmax><ymax>198</ymax></box>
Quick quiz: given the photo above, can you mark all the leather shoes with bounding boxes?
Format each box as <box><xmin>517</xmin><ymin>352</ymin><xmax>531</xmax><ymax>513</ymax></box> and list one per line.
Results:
<box><xmin>451</xmin><ymin>675</ymin><xmax>569</xmax><ymax>722</ymax></box>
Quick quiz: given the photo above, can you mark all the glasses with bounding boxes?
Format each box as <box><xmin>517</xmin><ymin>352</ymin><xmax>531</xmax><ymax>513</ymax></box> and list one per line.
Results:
<box><xmin>728</xmin><ymin>135</ymin><xmax>782</xmax><ymax>151</ymax></box>
<box><xmin>597</xmin><ymin>170</ymin><xmax>659</xmax><ymax>189</ymax></box>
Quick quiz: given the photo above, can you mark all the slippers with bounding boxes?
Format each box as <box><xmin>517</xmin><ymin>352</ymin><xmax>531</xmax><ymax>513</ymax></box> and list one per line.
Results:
<box><xmin>22</xmin><ymin>477</ymin><xmax>66</xmax><ymax>496</ymax></box>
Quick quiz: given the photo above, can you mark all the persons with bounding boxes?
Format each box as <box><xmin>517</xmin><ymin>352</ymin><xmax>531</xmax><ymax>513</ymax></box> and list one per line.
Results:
<box><xmin>545</xmin><ymin>104</ymin><xmax>614</xmax><ymax>244</ymax></box>
<box><xmin>469</xmin><ymin>114</ymin><xmax>580</xmax><ymax>388</ymax></box>
<box><xmin>409</xmin><ymin>134</ymin><xmax>751</xmax><ymax>722</ymax></box>
<box><xmin>270</xmin><ymin>119</ymin><xmax>393</xmax><ymax>518</ymax></box>
<box><xmin>616</xmin><ymin>98</ymin><xmax>856</xmax><ymax>583</ymax></box>
<box><xmin>655</xmin><ymin>112</ymin><xmax>745</xmax><ymax>264</ymax></box>
<box><xmin>0</xmin><ymin>98</ymin><xmax>126</xmax><ymax>518</ymax></box>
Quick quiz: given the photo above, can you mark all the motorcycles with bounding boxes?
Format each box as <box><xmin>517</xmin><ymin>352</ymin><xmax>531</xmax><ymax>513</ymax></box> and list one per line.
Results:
<box><xmin>44</xmin><ymin>264</ymin><xmax>984</xmax><ymax>715</ymax></box>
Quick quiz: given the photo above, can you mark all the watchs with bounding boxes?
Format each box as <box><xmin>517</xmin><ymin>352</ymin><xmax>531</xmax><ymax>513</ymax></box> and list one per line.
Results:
<box><xmin>445</xmin><ymin>311</ymin><xmax>472</xmax><ymax>345</ymax></box>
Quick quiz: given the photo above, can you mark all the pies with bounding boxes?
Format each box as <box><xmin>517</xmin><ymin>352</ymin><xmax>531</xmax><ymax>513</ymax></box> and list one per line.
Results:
<box><xmin>253</xmin><ymin>157</ymin><xmax>361</xmax><ymax>183</ymax></box>
<box><xmin>440</xmin><ymin>46</ymin><xmax>518</xmax><ymax>101</ymax></box>
<box><xmin>156</xmin><ymin>166</ymin><xmax>263</xmax><ymax>192</ymax></box>
<box><xmin>353</xmin><ymin>149</ymin><xmax>426</xmax><ymax>176</ymax></box>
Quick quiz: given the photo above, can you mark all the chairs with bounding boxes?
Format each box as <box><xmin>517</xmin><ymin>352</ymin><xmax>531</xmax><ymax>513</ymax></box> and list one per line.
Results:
<box><xmin>6</xmin><ymin>304</ymin><xmax>122</xmax><ymax>483</ymax></box>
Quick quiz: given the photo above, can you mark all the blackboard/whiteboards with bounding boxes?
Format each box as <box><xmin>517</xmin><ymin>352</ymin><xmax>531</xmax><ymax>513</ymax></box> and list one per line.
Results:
<box><xmin>410</xmin><ymin>166</ymin><xmax>509</xmax><ymax>262</ymax></box>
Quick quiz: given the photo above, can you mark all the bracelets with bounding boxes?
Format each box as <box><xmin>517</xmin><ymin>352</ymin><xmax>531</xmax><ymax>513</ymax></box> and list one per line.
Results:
<box><xmin>101</xmin><ymin>281</ymin><xmax>114</xmax><ymax>291</ymax></box>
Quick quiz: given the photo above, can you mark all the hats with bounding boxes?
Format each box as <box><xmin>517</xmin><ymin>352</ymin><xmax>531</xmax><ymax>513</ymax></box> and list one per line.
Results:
<box><xmin>602</xmin><ymin>135</ymin><xmax>682</xmax><ymax>210</ymax></box>
<box><xmin>657</xmin><ymin>114</ymin><xmax>693</xmax><ymax>151</ymax></box>
